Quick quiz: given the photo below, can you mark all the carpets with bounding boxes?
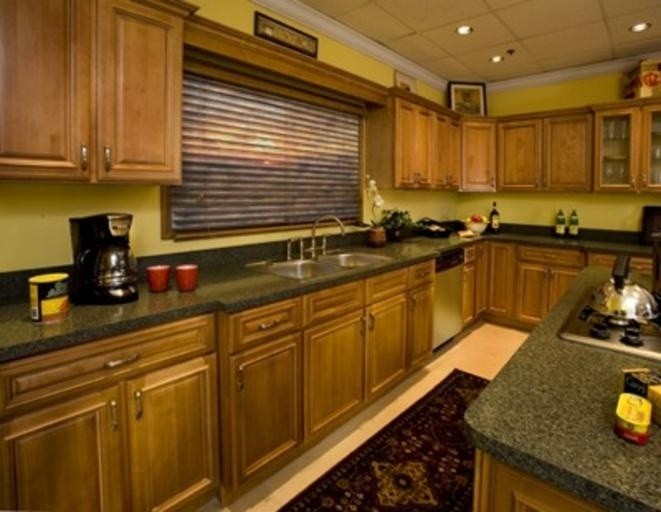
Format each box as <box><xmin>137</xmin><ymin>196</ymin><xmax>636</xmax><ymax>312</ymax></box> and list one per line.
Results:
<box><xmin>274</xmin><ymin>366</ymin><xmax>490</xmax><ymax>512</ymax></box>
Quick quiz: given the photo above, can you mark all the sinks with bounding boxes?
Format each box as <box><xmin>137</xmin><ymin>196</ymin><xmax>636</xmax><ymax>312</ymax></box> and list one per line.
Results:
<box><xmin>243</xmin><ymin>256</ymin><xmax>342</xmax><ymax>280</ymax></box>
<box><xmin>317</xmin><ymin>252</ymin><xmax>392</xmax><ymax>269</ymax></box>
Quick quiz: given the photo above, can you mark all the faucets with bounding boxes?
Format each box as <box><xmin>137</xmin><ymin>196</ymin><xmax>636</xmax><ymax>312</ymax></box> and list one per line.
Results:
<box><xmin>287</xmin><ymin>237</ymin><xmax>294</xmax><ymax>260</ymax></box>
<box><xmin>311</xmin><ymin>214</ymin><xmax>345</xmax><ymax>260</ymax></box>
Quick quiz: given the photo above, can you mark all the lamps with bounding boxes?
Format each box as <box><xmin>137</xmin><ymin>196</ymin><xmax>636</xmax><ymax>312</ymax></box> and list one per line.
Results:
<box><xmin>627</xmin><ymin>22</ymin><xmax>652</xmax><ymax>33</ymax></box>
<box><xmin>456</xmin><ymin>26</ymin><xmax>474</xmax><ymax>35</ymax></box>
<box><xmin>491</xmin><ymin>56</ymin><xmax>504</xmax><ymax>64</ymax></box>
<box><xmin>366</xmin><ymin>179</ymin><xmax>385</xmax><ymax>245</ymax></box>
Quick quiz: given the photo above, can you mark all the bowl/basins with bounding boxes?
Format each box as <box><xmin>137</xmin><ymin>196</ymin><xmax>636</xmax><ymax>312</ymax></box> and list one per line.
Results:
<box><xmin>466</xmin><ymin>222</ymin><xmax>486</xmax><ymax>234</ymax></box>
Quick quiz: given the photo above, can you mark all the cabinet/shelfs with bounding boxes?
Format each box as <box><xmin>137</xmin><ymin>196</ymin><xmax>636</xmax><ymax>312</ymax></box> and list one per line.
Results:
<box><xmin>0</xmin><ymin>254</ymin><xmax>221</xmax><ymax>512</ymax></box>
<box><xmin>1</xmin><ymin>2</ymin><xmax>199</xmax><ymax>187</ymax></box>
<box><xmin>248</xmin><ymin>235</ymin><xmax>420</xmax><ymax>453</ymax></box>
<box><xmin>450</xmin><ymin>218</ymin><xmax>488</xmax><ymax>328</ymax></box>
<box><xmin>588</xmin><ymin>247</ymin><xmax>661</xmax><ymax>274</ymax></box>
<box><xmin>369</xmin><ymin>228</ymin><xmax>437</xmax><ymax>374</ymax></box>
<box><xmin>594</xmin><ymin>96</ymin><xmax>661</xmax><ymax>195</ymax></box>
<box><xmin>149</xmin><ymin>237</ymin><xmax>302</xmax><ymax>507</ymax></box>
<box><xmin>367</xmin><ymin>86</ymin><xmax>438</xmax><ymax>192</ymax></box>
<box><xmin>463</xmin><ymin>116</ymin><xmax>497</xmax><ymax>194</ymax></box>
<box><xmin>516</xmin><ymin>229</ymin><xmax>586</xmax><ymax>333</ymax></box>
<box><xmin>497</xmin><ymin>110</ymin><xmax>594</xmax><ymax>193</ymax></box>
<box><xmin>470</xmin><ymin>444</ymin><xmax>615</xmax><ymax>512</ymax></box>
<box><xmin>438</xmin><ymin>103</ymin><xmax>462</xmax><ymax>194</ymax></box>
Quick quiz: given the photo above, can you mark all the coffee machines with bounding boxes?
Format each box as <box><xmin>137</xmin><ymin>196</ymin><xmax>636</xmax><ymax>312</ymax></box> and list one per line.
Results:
<box><xmin>69</xmin><ymin>213</ymin><xmax>139</xmax><ymax>306</ymax></box>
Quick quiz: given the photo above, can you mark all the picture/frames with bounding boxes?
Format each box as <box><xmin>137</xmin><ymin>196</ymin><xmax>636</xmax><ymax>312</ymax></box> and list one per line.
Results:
<box><xmin>447</xmin><ymin>80</ymin><xmax>486</xmax><ymax>116</ymax></box>
<box><xmin>254</xmin><ymin>9</ymin><xmax>325</xmax><ymax>58</ymax></box>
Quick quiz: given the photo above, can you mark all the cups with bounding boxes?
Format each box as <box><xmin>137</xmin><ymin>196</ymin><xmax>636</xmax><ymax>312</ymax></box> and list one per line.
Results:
<box><xmin>146</xmin><ymin>264</ymin><xmax>170</xmax><ymax>293</ymax></box>
<box><xmin>175</xmin><ymin>263</ymin><xmax>198</xmax><ymax>293</ymax></box>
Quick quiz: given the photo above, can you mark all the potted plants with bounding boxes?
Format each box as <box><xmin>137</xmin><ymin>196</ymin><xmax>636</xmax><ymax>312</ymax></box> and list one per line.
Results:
<box><xmin>378</xmin><ymin>208</ymin><xmax>421</xmax><ymax>240</ymax></box>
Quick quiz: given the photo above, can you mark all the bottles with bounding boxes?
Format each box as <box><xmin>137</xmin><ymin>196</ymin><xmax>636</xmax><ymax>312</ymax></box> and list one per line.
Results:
<box><xmin>555</xmin><ymin>208</ymin><xmax>565</xmax><ymax>238</ymax></box>
<box><xmin>489</xmin><ymin>201</ymin><xmax>499</xmax><ymax>234</ymax></box>
<box><xmin>568</xmin><ymin>209</ymin><xmax>579</xmax><ymax>239</ymax></box>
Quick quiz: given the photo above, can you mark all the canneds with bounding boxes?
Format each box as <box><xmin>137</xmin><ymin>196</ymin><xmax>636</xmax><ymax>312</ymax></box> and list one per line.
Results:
<box><xmin>28</xmin><ymin>273</ymin><xmax>69</xmax><ymax>324</ymax></box>
